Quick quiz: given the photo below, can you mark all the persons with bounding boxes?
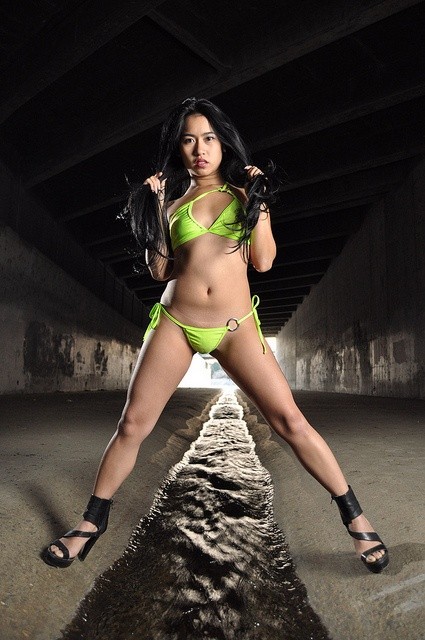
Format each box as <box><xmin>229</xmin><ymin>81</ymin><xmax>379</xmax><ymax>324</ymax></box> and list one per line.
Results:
<box><xmin>41</xmin><ymin>97</ymin><xmax>390</xmax><ymax>573</ymax></box>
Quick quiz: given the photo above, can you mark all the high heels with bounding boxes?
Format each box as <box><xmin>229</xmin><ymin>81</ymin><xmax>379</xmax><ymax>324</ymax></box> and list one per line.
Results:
<box><xmin>42</xmin><ymin>495</ymin><xmax>114</xmax><ymax>569</ymax></box>
<box><xmin>330</xmin><ymin>487</ymin><xmax>390</xmax><ymax>573</ymax></box>
